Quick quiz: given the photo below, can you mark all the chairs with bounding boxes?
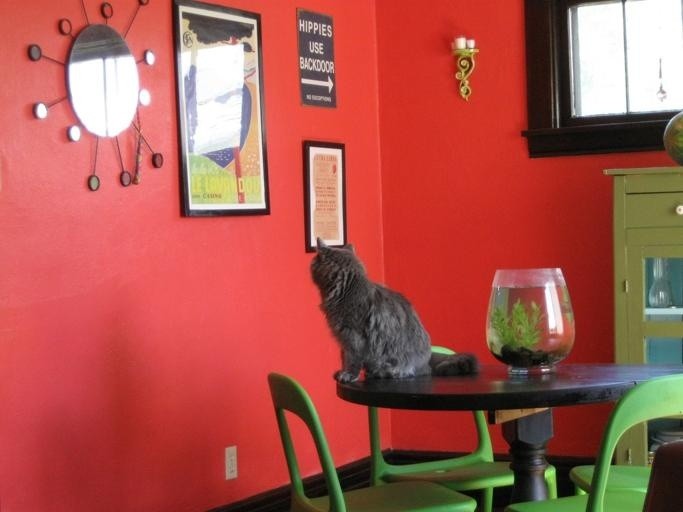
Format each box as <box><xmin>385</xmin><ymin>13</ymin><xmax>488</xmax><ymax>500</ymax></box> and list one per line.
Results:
<box><xmin>268</xmin><ymin>373</ymin><xmax>477</xmax><ymax>510</ymax></box>
<box><xmin>369</xmin><ymin>347</ymin><xmax>557</xmax><ymax>510</ymax></box>
<box><xmin>508</xmin><ymin>372</ymin><xmax>683</xmax><ymax>512</ymax></box>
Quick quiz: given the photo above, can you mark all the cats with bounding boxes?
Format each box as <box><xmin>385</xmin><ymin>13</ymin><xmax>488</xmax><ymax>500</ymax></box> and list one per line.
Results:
<box><xmin>308</xmin><ymin>236</ymin><xmax>478</xmax><ymax>385</ymax></box>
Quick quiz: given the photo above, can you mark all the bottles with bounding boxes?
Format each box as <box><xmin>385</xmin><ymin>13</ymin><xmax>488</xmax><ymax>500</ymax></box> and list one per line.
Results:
<box><xmin>649</xmin><ymin>258</ymin><xmax>673</xmax><ymax>309</ymax></box>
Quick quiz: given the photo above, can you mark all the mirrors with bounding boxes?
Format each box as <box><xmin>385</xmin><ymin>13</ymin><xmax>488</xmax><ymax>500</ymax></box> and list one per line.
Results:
<box><xmin>67</xmin><ymin>24</ymin><xmax>142</xmax><ymax>141</ymax></box>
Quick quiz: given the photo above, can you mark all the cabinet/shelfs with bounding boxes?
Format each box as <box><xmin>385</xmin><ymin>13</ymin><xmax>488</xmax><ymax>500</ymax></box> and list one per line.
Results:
<box><xmin>601</xmin><ymin>166</ymin><xmax>682</xmax><ymax>465</ymax></box>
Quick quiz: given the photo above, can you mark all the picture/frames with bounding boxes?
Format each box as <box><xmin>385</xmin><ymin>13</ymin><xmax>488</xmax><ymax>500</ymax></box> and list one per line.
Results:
<box><xmin>169</xmin><ymin>0</ymin><xmax>270</xmax><ymax>217</ymax></box>
<box><xmin>302</xmin><ymin>140</ymin><xmax>347</xmax><ymax>253</ymax></box>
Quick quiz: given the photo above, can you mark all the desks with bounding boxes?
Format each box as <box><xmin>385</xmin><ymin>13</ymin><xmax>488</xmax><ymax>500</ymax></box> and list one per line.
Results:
<box><xmin>338</xmin><ymin>363</ymin><xmax>682</xmax><ymax>503</ymax></box>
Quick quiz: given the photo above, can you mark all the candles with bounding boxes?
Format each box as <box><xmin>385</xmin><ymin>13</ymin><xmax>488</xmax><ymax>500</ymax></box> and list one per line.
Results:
<box><xmin>451</xmin><ymin>36</ymin><xmax>475</xmax><ymax>50</ymax></box>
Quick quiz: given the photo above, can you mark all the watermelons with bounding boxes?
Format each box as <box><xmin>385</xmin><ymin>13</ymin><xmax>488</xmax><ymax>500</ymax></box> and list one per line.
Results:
<box><xmin>663</xmin><ymin>111</ymin><xmax>683</xmax><ymax>164</ymax></box>
